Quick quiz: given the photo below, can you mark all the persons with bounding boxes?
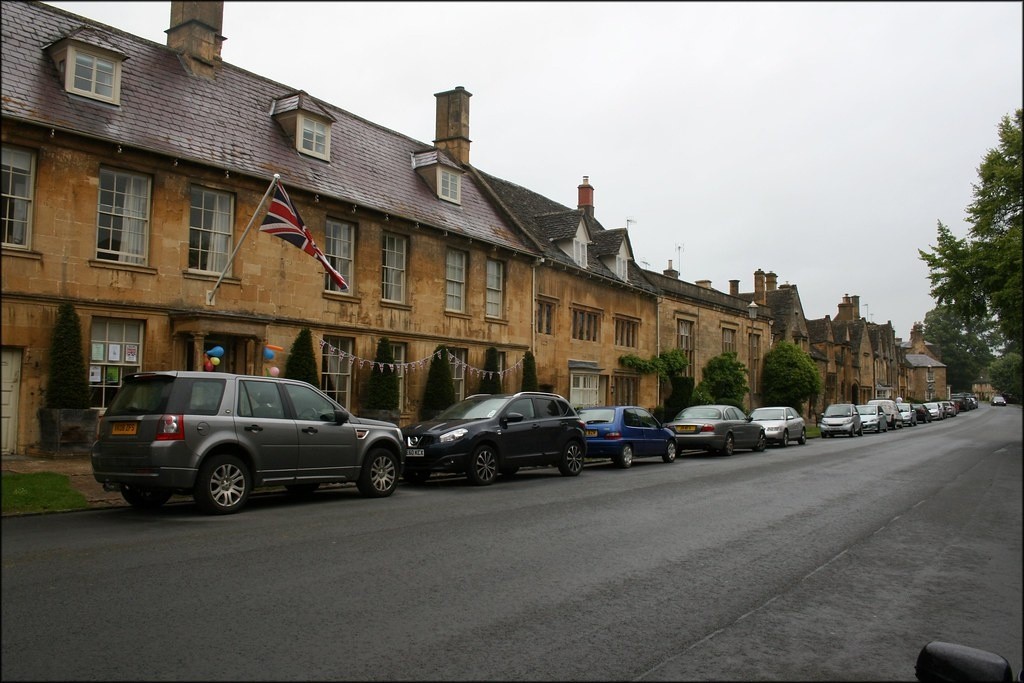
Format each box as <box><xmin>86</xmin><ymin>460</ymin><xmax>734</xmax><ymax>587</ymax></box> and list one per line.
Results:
<box><xmin>896</xmin><ymin>396</ymin><xmax>902</xmax><ymax>403</ymax></box>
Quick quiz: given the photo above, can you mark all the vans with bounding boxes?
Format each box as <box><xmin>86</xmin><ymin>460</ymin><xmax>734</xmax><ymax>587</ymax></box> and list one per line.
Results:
<box><xmin>868</xmin><ymin>400</ymin><xmax>904</xmax><ymax>429</ymax></box>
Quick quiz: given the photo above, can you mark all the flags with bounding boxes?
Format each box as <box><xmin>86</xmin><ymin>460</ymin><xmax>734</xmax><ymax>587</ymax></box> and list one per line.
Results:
<box><xmin>259</xmin><ymin>179</ymin><xmax>349</xmax><ymax>290</ymax></box>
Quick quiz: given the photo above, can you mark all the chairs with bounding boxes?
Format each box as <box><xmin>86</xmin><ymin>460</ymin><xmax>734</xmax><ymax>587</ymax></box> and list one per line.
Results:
<box><xmin>251</xmin><ymin>387</ymin><xmax>280</xmax><ymax>418</ymax></box>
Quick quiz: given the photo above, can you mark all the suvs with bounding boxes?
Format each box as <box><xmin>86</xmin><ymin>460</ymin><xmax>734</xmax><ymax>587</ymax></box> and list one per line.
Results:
<box><xmin>90</xmin><ymin>370</ymin><xmax>407</xmax><ymax>514</ymax></box>
<box><xmin>400</xmin><ymin>391</ymin><xmax>588</xmax><ymax>486</ymax></box>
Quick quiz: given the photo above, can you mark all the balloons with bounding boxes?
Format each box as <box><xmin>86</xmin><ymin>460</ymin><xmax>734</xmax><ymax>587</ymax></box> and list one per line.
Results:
<box><xmin>204</xmin><ymin>354</ymin><xmax>214</xmax><ymax>371</ymax></box>
<box><xmin>264</xmin><ymin>348</ymin><xmax>274</xmax><ymax>359</ymax></box>
<box><xmin>207</xmin><ymin>346</ymin><xmax>224</xmax><ymax>356</ymax></box>
<box><xmin>266</xmin><ymin>345</ymin><xmax>283</xmax><ymax>351</ymax></box>
<box><xmin>266</xmin><ymin>367</ymin><xmax>280</xmax><ymax>377</ymax></box>
<box><xmin>210</xmin><ymin>357</ymin><xmax>220</xmax><ymax>365</ymax></box>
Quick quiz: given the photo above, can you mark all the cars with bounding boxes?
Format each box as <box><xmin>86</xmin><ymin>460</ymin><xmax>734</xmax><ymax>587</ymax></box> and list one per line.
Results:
<box><xmin>661</xmin><ymin>405</ymin><xmax>768</xmax><ymax>458</ymax></box>
<box><xmin>572</xmin><ymin>406</ymin><xmax>679</xmax><ymax>469</ymax></box>
<box><xmin>855</xmin><ymin>405</ymin><xmax>889</xmax><ymax>433</ymax></box>
<box><xmin>940</xmin><ymin>392</ymin><xmax>979</xmax><ymax>417</ymax></box>
<box><xmin>895</xmin><ymin>403</ymin><xmax>918</xmax><ymax>427</ymax></box>
<box><xmin>746</xmin><ymin>407</ymin><xmax>807</xmax><ymax>447</ymax></box>
<box><xmin>990</xmin><ymin>396</ymin><xmax>1007</xmax><ymax>406</ymax></box>
<box><xmin>925</xmin><ymin>403</ymin><xmax>944</xmax><ymax>420</ymax></box>
<box><xmin>820</xmin><ymin>403</ymin><xmax>864</xmax><ymax>437</ymax></box>
<box><xmin>937</xmin><ymin>402</ymin><xmax>948</xmax><ymax>419</ymax></box>
<box><xmin>911</xmin><ymin>403</ymin><xmax>933</xmax><ymax>423</ymax></box>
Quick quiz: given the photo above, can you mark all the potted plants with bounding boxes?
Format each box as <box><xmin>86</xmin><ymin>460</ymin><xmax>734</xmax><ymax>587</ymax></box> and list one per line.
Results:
<box><xmin>39</xmin><ymin>302</ymin><xmax>99</xmax><ymax>452</ymax></box>
<box><xmin>419</xmin><ymin>345</ymin><xmax>456</xmax><ymax>422</ymax></box>
<box><xmin>359</xmin><ymin>335</ymin><xmax>401</xmax><ymax>429</ymax></box>
